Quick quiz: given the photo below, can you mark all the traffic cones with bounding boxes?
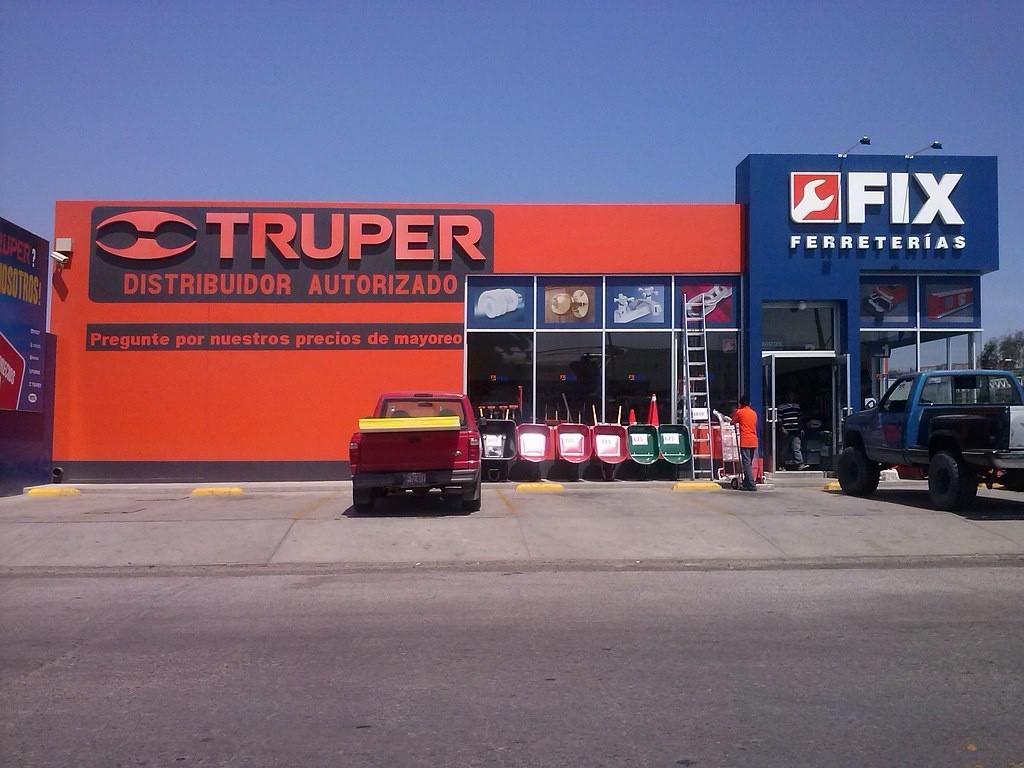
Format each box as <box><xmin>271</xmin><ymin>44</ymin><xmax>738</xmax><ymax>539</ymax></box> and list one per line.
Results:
<box><xmin>646</xmin><ymin>393</ymin><xmax>660</xmax><ymax>428</ymax></box>
<box><xmin>628</xmin><ymin>408</ymin><xmax>637</xmax><ymax>425</ymax></box>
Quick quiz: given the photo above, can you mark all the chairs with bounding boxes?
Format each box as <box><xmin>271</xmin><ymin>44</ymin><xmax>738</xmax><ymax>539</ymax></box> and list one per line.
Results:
<box><xmin>438</xmin><ymin>408</ymin><xmax>457</xmax><ymax>416</ymax></box>
<box><xmin>391</xmin><ymin>410</ymin><xmax>410</xmax><ymax>418</ymax></box>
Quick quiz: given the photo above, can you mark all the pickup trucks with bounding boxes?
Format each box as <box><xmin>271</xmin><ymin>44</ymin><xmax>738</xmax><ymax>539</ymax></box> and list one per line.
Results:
<box><xmin>347</xmin><ymin>391</ymin><xmax>483</xmax><ymax>511</ymax></box>
<box><xmin>838</xmin><ymin>370</ymin><xmax>1024</xmax><ymax>509</ymax></box>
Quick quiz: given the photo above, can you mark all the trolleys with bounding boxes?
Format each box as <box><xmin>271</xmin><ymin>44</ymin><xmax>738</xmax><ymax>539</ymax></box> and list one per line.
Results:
<box><xmin>480</xmin><ymin>405</ymin><xmax>692</xmax><ymax>481</ymax></box>
<box><xmin>731</xmin><ymin>420</ymin><xmax>744</xmax><ymax>490</ymax></box>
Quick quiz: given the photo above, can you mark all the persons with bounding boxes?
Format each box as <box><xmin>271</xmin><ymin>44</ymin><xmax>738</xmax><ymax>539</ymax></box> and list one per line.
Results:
<box><xmin>778</xmin><ymin>391</ymin><xmax>810</xmax><ymax>471</ymax></box>
<box><xmin>731</xmin><ymin>395</ymin><xmax>759</xmax><ymax>491</ymax></box>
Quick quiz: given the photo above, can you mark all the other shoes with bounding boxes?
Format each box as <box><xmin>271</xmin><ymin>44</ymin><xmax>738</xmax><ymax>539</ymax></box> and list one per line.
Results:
<box><xmin>748</xmin><ymin>486</ymin><xmax>756</xmax><ymax>490</ymax></box>
<box><xmin>798</xmin><ymin>464</ymin><xmax>804</xmax><ymax>470</ymax></box>
<box><xmin>779</xmin><ymin>467</ymin><xmax>786</xmax><ymax>471</ymax></box>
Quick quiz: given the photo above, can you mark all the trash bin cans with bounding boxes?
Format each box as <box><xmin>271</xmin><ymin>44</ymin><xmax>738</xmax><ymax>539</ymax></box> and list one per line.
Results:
<box><xmin>617</xmin><ymin>425</ymin><xmax>659</xmax><ymax>482</ymax></box>
<box><xmin>476</xmin><ymin>418</ymin><xmax>517</xmax><ymax>481</ymax></box>
<box><xmin>653</xmin><ymin>424</ymin><xmax>691</xmax><ymax>480</ymax></box>
<box><xmin>584</xmin><ymin>424</ymin><xmax>629</xmax><ymax>481</ymax></box>
<box><xmin>818</xmin><ymin>446</ymin><xmax>833</xmax><ymax>470</ymax></box>
<box><xmin>547</xmin><ymin>423</ymin><xmax>592</xmax><ymax>481</ymax></box>
<box><xmin>508</xmin><ymin>423</ymin><xmax>550</xmax><ymax>482</ymax></box>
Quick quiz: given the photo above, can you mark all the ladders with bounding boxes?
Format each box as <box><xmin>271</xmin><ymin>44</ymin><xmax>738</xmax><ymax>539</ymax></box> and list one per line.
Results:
<box><xmin>682</xmin><ymin>293</ymin><xmax>714</xmax><ymax>481</ymax></box>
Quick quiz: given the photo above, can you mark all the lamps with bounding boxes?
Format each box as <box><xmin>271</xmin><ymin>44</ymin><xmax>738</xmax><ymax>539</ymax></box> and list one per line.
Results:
<box><xmin>905</xmin><ymin>141</ymin><xmax>944</xmax><ymax>159</ymax></box>
<box><xmin>838</xmin><ymin>136</ymin><xmax>871</xmax><ymax>158</ymax></box>
